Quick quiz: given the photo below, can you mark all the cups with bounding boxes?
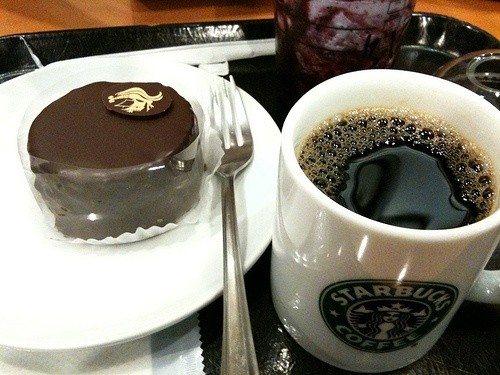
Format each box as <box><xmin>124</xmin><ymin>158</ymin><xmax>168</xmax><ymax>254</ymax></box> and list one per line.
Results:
<box><xmin>269</xmin><ymin>67</ymin><xmax>500</xmax><ymax>372</ymax></box>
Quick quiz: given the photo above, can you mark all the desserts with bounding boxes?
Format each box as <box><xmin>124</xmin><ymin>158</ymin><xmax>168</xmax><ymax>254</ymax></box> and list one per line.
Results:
<box><xmin>25</xmin><ymin>82</ymin><xmax>202</xmax><ymax>241</ymax></box>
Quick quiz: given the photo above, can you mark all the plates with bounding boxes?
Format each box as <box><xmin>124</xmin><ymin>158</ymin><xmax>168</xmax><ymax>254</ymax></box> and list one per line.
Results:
<box><xmin>2</xmin><ymin>53</ymin><xmax>282</xmax><ymax>353</ymax></box>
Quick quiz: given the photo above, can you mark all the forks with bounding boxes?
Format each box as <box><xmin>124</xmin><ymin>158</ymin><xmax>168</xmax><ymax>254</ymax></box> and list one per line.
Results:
<box><xmin>199</xmin><ymin>75</ymin><xmax>260</xmax><ymax>374</ymax></box>
